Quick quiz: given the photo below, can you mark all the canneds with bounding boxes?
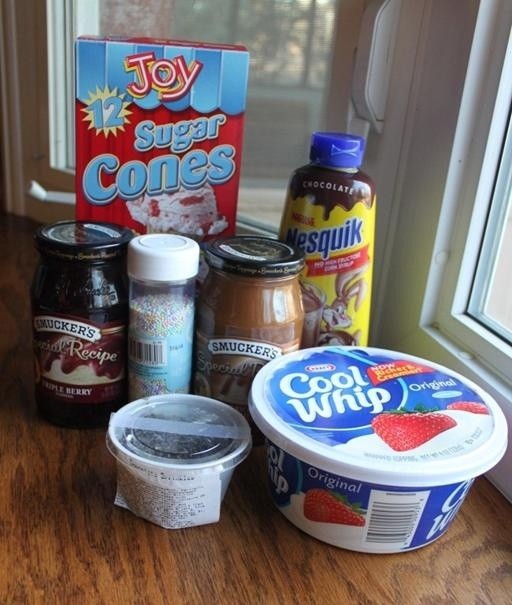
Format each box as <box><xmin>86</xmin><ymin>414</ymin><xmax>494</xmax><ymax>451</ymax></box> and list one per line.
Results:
<box><xmin>29</xmin><ymin>219</ymin><xmax>128</xmax><ymax>428</ymax></box>
<box><xmin>194</xmin><ymin>234</ymin><xmax>306</xmax><ymax>446</ymax></box>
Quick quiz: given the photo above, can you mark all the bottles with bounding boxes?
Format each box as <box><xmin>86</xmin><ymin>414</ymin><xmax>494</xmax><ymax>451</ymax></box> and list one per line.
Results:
<box><xmin>126</xmin><ymin>230</ymin><xmax>201</xmax><ymax>402</ymax></box>
<box><xmin>276</xmin><ymin>130</ymin><xmax>377</xmax><ymax>349</ymax></box>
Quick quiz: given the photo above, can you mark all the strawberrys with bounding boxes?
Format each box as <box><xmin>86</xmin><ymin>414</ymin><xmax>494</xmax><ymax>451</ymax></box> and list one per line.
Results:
<box><xmin>448</xmin><ymin>402</ymin><xmax>488</xmax><ymax>414</ymax></box>
<box><xmin>303</xmin><ymin>488</ymin><xmax>365</xmax><ymax>526</ymax></box>
<box><xmin>371</xmin><ymin>410</ymin><xmax>456</xmax><ymax>451</ymax></box>
